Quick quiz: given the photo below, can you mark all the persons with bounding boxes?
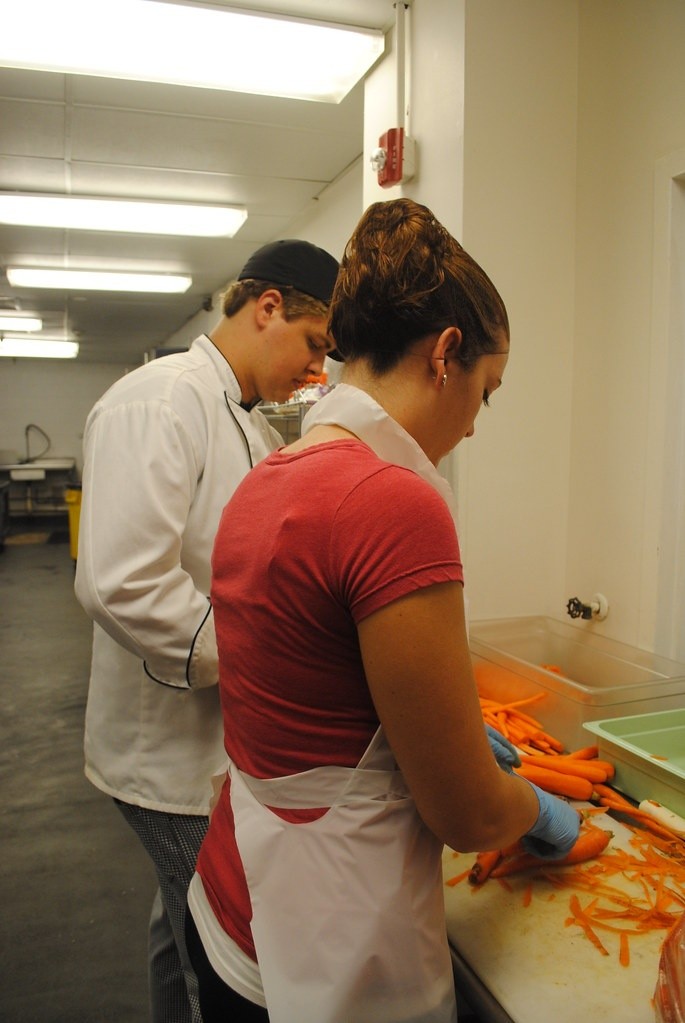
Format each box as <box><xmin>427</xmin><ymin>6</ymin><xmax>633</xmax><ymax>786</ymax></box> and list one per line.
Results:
<box><xmin>64</xmin><ymin>227</ymin><xmax>338</xmax><ymax>1022</ymax></box>
<box><xmin>179</xmin><ymin>192</ymin><xmax>586</xmax><ymax>1023</ymax></box>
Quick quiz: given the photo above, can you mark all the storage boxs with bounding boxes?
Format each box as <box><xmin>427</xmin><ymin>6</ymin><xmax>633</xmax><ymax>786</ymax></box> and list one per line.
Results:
<box><xmin>64</xmin><ymin>480</ymin><xmax>83</xmax><ymax>560</ymax></box>
<box><xmin>576</xmin><ymin>707</ymin><xmax>685</xmax><ymax>818</ymax></box>
<box><xmin>466</xmin><ymin>613</ymin><xmax>685</xmax><ymax>751</ymax></box>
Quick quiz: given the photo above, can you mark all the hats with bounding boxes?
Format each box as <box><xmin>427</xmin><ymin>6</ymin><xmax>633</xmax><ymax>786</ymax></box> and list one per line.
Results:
<box><xmin>237</xmin><ymin>238</ymin><xmax>347</xmax><ymax>362</ymax></box>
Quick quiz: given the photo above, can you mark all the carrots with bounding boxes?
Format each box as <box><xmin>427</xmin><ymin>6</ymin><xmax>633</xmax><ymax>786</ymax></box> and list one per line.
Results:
<box><xmin>470</xmin><ymin>694</ymin><xmax>683</xmax><ymax>1023</ymax></box>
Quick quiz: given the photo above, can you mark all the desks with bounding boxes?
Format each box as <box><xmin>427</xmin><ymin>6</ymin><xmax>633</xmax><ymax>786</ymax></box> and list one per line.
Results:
<box><xmin>440</xmin><ymin>786</ymin><xmax>685</xmax><ymax>1022</ymax></box>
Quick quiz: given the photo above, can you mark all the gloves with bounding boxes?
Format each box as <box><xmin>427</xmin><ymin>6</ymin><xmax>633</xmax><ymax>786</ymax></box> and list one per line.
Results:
<box><xmin>484</xmin><ymin>722</ymin><xmax>521</xmax><ymax>774</ymax></box>
<box><xmin>510</xmin><ymin>771</ymin><xmax>580</xmax><ymax>862</ymax></box>
